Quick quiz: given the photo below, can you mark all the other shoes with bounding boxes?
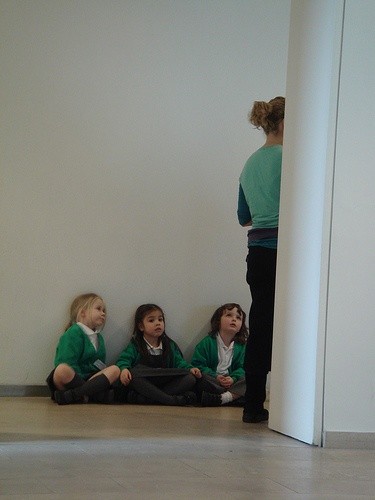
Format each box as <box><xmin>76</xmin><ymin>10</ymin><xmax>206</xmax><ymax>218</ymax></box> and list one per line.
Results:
<box><xmin>127</xmin><ymin>391</ymin><xmax>147</xmax><ymax>404</ymax></box>
<box><xmin>93</xmin><ymin>388</ymin><xmax>114</xmax><ymax>404</ymax></box>
<box><xmin>243</xmin><ymin>408</ymin><xmax>269</xmax><ymax>423</ymax></box>
<box><xmin>176</xmin><ymin>391</ymin><xmax>198</xmax><ymax>405</ymax></box>
<box><xmin>201</xmin><ymin>391</ymin><xmax>223</xmax><ymax>407</ymax></box>
<box><xmin>54</xmin><ymin>390</ymin><xmax>76</xmax><ymax>405</ymax></box>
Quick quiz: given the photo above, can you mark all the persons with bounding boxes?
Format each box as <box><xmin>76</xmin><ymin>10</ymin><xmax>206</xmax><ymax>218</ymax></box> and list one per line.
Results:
<box><xmin>190</xmin><ymin>303</ymin><xmax>249</xmax><ymax>408</ymax></box>
<box><xmin>237</xmin><ymin>95</ymin><xmax>286</xmax><ymax>422</ymax></box>
<box><xmin>110</xmin><ymin>303</ymin><xmax>202</xmax><ymax>406</ymax></box>
<box><xmin>46</xmin><ymin>293</ymin><xmax>121</xmax><ymax>406</ymax></box>
